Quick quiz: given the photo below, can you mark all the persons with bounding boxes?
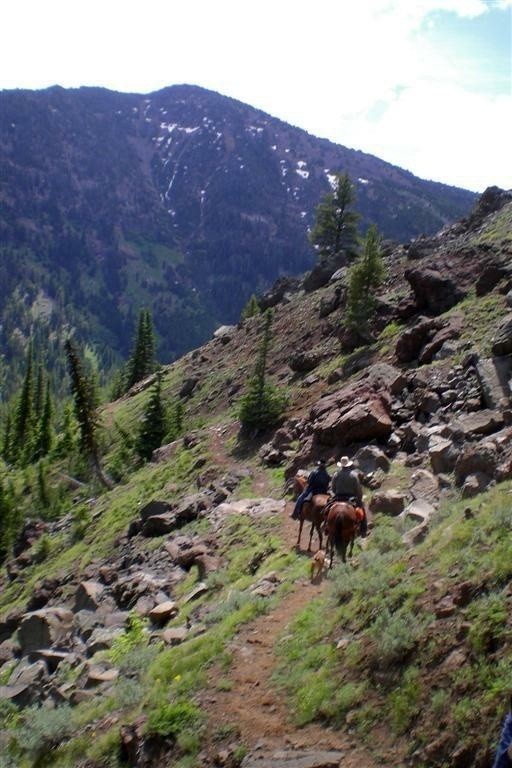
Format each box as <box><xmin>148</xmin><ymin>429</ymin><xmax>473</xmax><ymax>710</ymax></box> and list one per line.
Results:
<box><xmin>289</xmin><ymin>457</ymin><xmax>329</xmax><ymax>519</ymax></box>
<box><xmin>331</xmin><ymin>456</ymin><xmax>371</xmax><ymax>538</ymax></box>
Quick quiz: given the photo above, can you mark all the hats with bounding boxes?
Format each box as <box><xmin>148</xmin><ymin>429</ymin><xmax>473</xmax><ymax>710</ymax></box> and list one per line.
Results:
<box><xmin>337</xmin><ymin>455</ymin><xmax>354</xmax><ymax>468</ymax></box>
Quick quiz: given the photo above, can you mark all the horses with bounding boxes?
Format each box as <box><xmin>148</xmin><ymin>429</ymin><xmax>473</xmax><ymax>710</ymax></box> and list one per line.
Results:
<box><xmin>291</xmin><ymin>473</ymin><xmax>332</xmax><ymax>555</ymax></box>
<box><xmin>324</xmin><ymin>499</ymin><xmax>359</xmax><ymax>569</ymax></box>
